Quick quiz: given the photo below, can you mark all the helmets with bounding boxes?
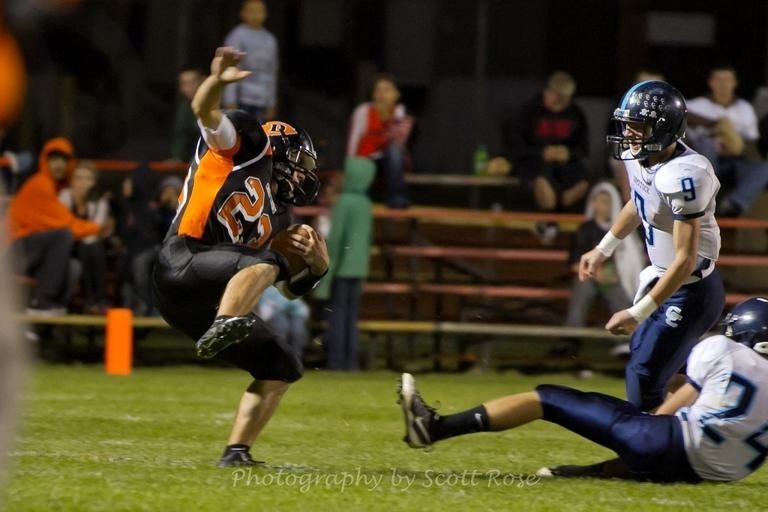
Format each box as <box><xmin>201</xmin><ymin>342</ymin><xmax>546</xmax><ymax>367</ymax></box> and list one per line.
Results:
<box><xmin>721</xmin><ymin>297</ymin><xmax>768</xmax><ymax>359</ymax></box>
<box><xmin>615</xmin><ymin>80</ymin><xmax>688</xmax><ymax>160</ymax></box>
<box><xmin>259</xmin><ymin>120</ymin><xmax>321</xmax><ymax>200</ymax></box>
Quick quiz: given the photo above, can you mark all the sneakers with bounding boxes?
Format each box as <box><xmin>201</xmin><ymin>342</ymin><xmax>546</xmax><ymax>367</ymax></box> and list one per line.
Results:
<box><xmin>22</xmin><ymin>298</ymin><xmax>69</xmax><ymax>318</ymax></box>
<box><xmin>396</xmin><ymin>372</ymin><xmax>435</xmax><ymax>449</ymax></box>
<box><xmin>195</xmin><ymin>315</ymin><xmax>258</xmax><ymax>361</ymax></box>
<box><xmin>216</xmin><ymin>444</ymin><xmax>265</xmax><ymax>469</ymax></box>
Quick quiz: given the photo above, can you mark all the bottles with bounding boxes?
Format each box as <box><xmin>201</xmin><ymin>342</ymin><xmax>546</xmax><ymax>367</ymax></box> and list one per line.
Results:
<box><xmin>473</xmin><ymin>145</ymin><xmax>487</xmax><ymax>175</ymax></box>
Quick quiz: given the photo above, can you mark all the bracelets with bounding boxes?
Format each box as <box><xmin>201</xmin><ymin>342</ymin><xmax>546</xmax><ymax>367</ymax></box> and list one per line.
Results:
<box><xmin>625</xmin><ymin>294</ymin><xmax>659</xmax><ymax>325</ymax></box>
<box><xmin>286</xmin><ymin>265</ymin><xmax>329</xmax><ymax>296</ymax></box>
<box><xmin>595</xmin><ymin>230</ymin><xmax>623</xmax><ymax>260</ymax></box>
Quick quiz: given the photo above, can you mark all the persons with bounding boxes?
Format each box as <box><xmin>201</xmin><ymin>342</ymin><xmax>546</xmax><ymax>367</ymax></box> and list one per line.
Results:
<box><xmin>399</xmin><ymin>296</ymin><xmax>768</xmax><ymax>482</ymax></box>
<box><xmin>169</xmin><ymin>67</ymin><xmax>203</xmax><ymax>156</ymax></box>
<box><xmin>510</xmin><ymin>68</ymin><xmax>592</xmax><ymax>212</ymax></box>
<box><xmin>130</xmin><ymin>175</ymin><xmax>182</xmax><ymax>288</ymax></box>
<box><xmin>682</xmin><ymin>68</ymin><xmax>768</xmax><ymax>216</ymax></box>
<box><xmin>2</xmin><ymin>137</ymin><xmax>107</xmax><ymax>309</ymax></box>
<box><xmin>220</xmin><ymin>0</ymin><xmax>280</xmax><ymax>115</ymax></box>
<box><xmin>59</xmin><ymin>164</ymin><xmax>111</xmax><ymax>312</ymax></box>
<box><xmin>568</xmin><ymin>183</ymin><xmax>645</xmax><ymax>335</ymax></box>
<box><xmin>149</xmin><ymin>45</ymin><xmax>331</xmax><ymax>465</ymax></box>
<box><xmin>606</xmin><ymin>68</ymin><xmax>667</xmax><ymax>202</ymax></box>
<box><xmin>313</xmin><ymin>156</ymin><xmax>377</xmax><ymax>371</ymax></box>
<box><xmin>579</xmin><ymin>77</ymin><xmax>725</xmax><ymax>419</ymax></box>
<box><xmin>343</xmin><ymin>78</ymin><xmax>413</xmax><ymax>209</ymax></box>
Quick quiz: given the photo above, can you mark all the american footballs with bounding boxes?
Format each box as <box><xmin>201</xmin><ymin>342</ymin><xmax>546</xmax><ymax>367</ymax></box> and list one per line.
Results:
<box><xmin>264</xmin><ymin>223</ymin><xmax>317</xmax><ymax>276</ymax></box>
